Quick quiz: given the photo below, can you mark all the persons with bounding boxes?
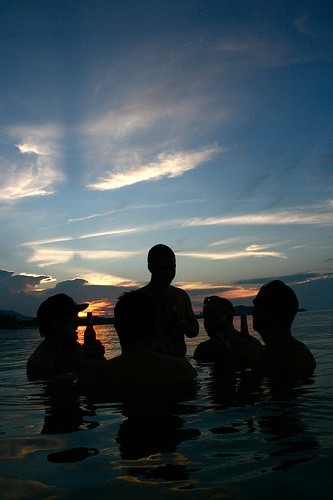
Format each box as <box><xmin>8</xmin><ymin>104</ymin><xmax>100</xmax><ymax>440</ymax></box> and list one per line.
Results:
<box><xmin>114</xmin><ymin>244</ymin><xmax>200</xmax><ymax>359</ymax></box>
<box><xmin>26</xmin><ymin>293</ymin><xmax>104</xmax><ymax>380</ymax></box>
<box><xmin>80</xmin><ymin>292</ymin><xmax>196</xmax><ymax>401</ymax></box>
<box><xmin>236</xmin><ymin>281</ymin><xmax>316</xmax><ymax>378</ymax></box>
<box><xmin>193</xmin><ymin>296</ymin><xmax>262</xmax><ymax>363</ymax></box>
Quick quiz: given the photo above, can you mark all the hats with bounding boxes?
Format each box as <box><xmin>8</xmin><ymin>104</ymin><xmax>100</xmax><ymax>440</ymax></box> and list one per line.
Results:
<box><xmin>37</xmin><ymin>294</ymin><xmax>89</xmax><ymax>323</ymax></box>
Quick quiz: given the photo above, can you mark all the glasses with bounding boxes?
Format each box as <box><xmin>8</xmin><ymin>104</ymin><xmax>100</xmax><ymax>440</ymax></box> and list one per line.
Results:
<box><xmin>204</xmin><ymin>296</ymin><xmax>223</xmax><ymax>305</ymax></box>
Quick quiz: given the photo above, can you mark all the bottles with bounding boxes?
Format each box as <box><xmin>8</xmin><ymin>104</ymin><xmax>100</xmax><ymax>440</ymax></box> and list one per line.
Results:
<box><xmin>83</xmin><ymin>311</ymin><xmax>96</xmax><ymax>347</ymax></box>
<box><xmin>241</xmin><ymin>312</ymin><xmax>248</xmax><ymax>334</ymax></box>
